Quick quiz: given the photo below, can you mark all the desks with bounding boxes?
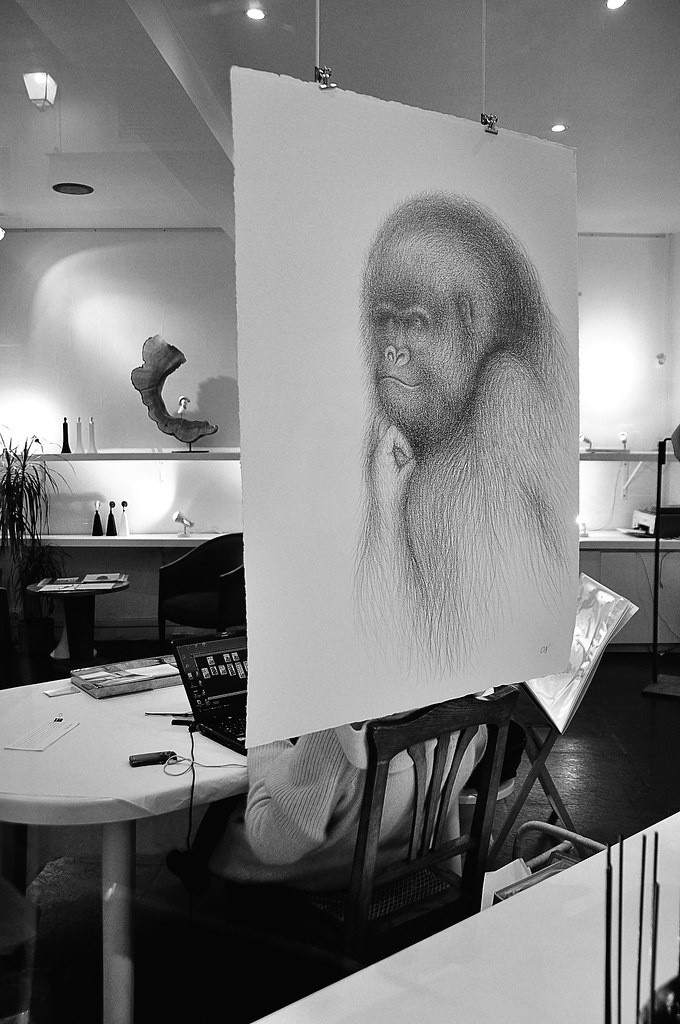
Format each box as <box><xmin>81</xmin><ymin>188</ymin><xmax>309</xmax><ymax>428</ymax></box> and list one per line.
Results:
<box><xmin>26</xmin><ymin>580</ymin><xmax>130</xmax><ymax>668</ymax></box>
<box><xmin>246</xmin><ymin>809</ymin><xmax>680</xmax><ymax>1024</ymax></box>
<box><xmin>0</xmin><ymin>677</ymin><xmax>249</xmax><ymax>1024</ymax></box>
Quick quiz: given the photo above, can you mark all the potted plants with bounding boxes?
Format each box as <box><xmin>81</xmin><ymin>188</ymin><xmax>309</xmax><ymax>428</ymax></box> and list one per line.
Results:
<box><xmin>0</xmin><ymin>434</ymin><xmax>76</xmax><ymax>655</ymax></box>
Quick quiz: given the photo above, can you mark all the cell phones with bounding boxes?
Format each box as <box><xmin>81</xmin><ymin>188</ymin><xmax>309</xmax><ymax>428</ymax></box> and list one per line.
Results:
<box><xmin>130</xmin><ymin>751</ymin><xmax>177</xmax><ymax>767</ymax></box>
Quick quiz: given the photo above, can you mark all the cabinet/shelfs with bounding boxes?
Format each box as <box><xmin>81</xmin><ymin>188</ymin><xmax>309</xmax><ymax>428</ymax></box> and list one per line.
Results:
<box><xmin>0</xmin><ymin>451</ymin><xmax>680</xmax><ymax>652</ymax></box>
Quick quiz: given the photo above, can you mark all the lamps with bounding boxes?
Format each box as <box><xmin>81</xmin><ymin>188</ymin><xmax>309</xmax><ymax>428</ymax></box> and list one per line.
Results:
<box><xmin>576</xmin><ymin>518</ymin><xmax>588</xmax><ymax>537</ymax></box>
<box><xmin>578</xmin><ymin>433</ymin><xmax>594</xmax><ymax>451</ymax></box>
<box><xmin>171</xmin><ymin>512</ymin><xmax>194</xmax><ymax>538</ymax></box>
<box><xmin>22</xmin><ymin>70</ymin><xmax>57</xmax><ymax>112</ymax></box>
<box><xmin>619</xmin><ymin>432</ymin><xmax>627</xmax><ymax>452</ymax></box>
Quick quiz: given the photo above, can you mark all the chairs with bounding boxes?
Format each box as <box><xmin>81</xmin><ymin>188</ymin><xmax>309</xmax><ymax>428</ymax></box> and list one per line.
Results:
<box><xmin>222</xmin><ymin>685</ymin><xmax>520</xmax><ymax>1006</ymax></box>
<box><xmin>157</xmin><ymin>533</ymin><xmax>246</xmax><ymax>656</ymax></box>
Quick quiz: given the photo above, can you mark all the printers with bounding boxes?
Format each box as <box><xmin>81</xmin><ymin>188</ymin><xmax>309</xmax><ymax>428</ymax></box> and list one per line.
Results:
<box><xmin>617</xmin><ymin>505</ymin><xmax>680</xmax><ymax>539</ymax></box>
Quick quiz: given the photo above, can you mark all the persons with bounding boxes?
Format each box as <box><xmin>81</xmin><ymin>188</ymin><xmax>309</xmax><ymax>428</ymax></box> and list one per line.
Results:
<box><xmin>211</xmin><ymin>689</ymin><xmax>489</xmax><ymax>878</ymax></box>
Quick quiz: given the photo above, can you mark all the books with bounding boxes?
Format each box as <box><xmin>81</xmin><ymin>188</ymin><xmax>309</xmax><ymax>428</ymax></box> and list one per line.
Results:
<box><xmin>71</xmin><ymin>655</ymin><xmax>185</xmax><ymax>699</ymax></box>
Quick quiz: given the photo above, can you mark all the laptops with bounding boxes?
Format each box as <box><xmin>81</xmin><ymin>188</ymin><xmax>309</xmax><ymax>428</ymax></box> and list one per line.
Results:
<box><xmin>171</xmin><ymin>628</ymin><xmax>248</xmax><ymax>758</ymax></box>
